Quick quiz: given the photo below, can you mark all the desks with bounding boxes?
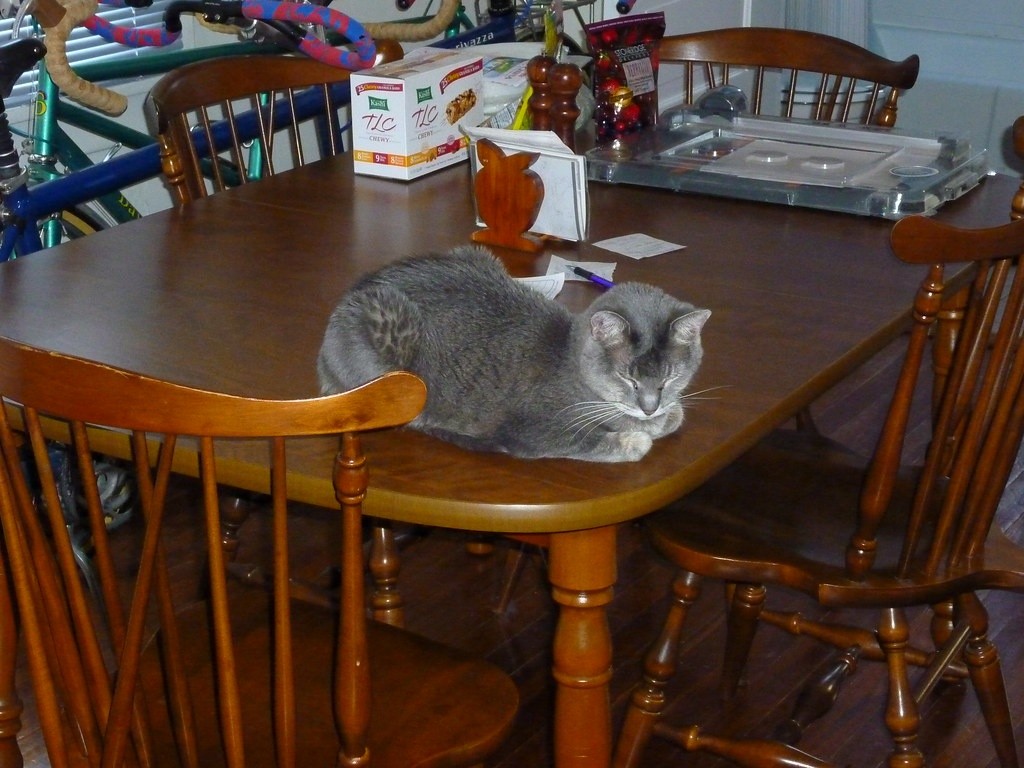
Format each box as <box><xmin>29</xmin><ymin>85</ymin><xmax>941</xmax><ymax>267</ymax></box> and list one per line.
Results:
<box><xmin>1</xmin><ymin>130</ymin><xmax>1024</xmax><ymax>768</ymax></box>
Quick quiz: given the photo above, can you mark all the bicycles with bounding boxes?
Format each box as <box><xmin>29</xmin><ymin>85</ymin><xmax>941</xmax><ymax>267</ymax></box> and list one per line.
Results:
<box><xmin>0</xmin><ymin>0</ymin><xmax>634</xmax><ymax>265</ymax></box>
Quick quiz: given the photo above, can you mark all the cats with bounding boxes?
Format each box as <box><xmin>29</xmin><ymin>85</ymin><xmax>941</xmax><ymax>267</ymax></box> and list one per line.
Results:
<box><xmin>317</xmin><ymin>244</ymin><xmax>712</xmax><ymax>464</ymax></box>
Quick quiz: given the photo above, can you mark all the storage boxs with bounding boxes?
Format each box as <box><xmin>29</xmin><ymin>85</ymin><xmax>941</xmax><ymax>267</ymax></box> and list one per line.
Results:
<box><xmin>350</xmin><ymin>52</ymin><xmax>485</xmax><ymax>180</ymax></box>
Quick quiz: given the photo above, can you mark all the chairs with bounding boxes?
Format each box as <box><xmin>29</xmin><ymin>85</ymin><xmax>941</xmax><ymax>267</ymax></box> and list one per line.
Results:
<box><xmin>143</xmin><ymin>54</ymin><xmax>492</xmax><ymax>631</ymax></box>
<box><xmin>613</xmin><ymin>116</ymin><xmax>1024</xmax><ymax>768</ymax></box>
<box><xmin>654</xmin><ymin>27</ymin><xmax>920</xmax><ymax>128</ymax></box>
<box><xmin>0</xmin><ymin>335</ymin><xmax>523</xmax><ymax>768</ymax></box>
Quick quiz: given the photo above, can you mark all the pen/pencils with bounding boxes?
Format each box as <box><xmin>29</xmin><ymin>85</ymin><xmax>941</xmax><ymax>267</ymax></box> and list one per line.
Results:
<box><xmin>564</xmin><ymin>264</ymin><xmax>617</xmax><ymax>288</ymax></box>
